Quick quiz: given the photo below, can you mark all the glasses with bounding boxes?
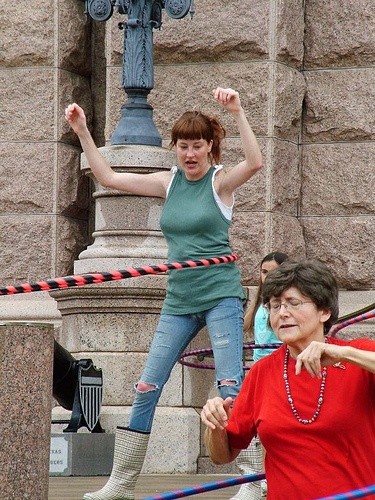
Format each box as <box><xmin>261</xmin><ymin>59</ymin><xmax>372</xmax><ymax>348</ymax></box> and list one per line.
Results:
<box><xmin>263</xmin><ymin>298</ymin><xmax>314</xmax><ymax>312</ymax></box>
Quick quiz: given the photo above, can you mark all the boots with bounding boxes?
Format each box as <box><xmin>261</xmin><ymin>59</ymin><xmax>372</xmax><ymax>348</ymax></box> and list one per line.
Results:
<box><xmin>81</xmin><ymin>426</ymin><xmax>151</xmax><ymax>499</ymax></box>
<box><xmin>230</xmin><ymin>436</ymin><xmax>262</xmax><ymax>500</ymax></box>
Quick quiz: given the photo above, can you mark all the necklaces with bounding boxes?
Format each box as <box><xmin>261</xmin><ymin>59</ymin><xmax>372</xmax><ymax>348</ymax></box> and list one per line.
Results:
<box><xmin>282</xmin><ymin>348</ymin><xmax>328</xmax><ymax>425</ymax></box>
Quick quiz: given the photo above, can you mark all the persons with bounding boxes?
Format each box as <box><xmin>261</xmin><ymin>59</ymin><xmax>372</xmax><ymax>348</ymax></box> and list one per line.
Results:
<box><xmin>242</xmin><ymin>249</ymin><xmax>290</xmax><ymax>369</ymax></box>
<box><xmin>64</xmin><ymin>84</ymin><xmax>263</xmax><ymax>500</ymax></box>
<box><xmin>201</xmin><ymin>258</ymin><xmax>375</xmax><ymax>500</ymax></box>
